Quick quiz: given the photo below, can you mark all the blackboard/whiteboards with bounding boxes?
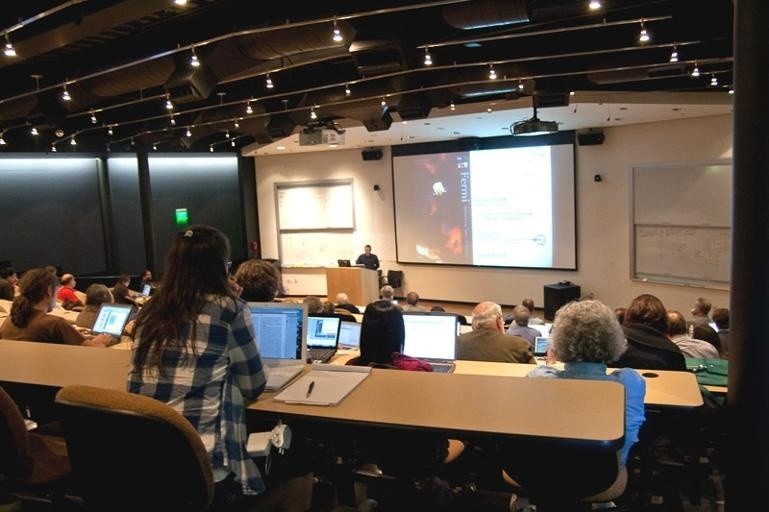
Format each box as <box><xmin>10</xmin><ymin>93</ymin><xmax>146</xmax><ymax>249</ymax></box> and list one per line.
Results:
<box><xmin>274</xmin><ymin>177</ymin><xmax>356</xmax><ymax>269</ymax></box>
<box><xmin>627</xmin><ymin>159</ymin><xmax>734</xmax><ymax>291</ymax></box>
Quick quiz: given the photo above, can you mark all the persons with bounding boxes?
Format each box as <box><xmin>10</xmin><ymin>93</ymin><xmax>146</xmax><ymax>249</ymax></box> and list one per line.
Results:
<box><xmin>283</xmin><ymin>286</ymin><xmax>446</xmax><ymax>323</ymax></box>
<box><xmin>355</xmin><ymin>245</ymin><xmax>380</xmax><ymax>270</ymax></box>
<box><xmin>530</xmin><ymin>298</ymin><xmax>646</xmax><ymax>511</ymax></box>
<box><xmin>127</xmin><ymin>223</ymin><xmax>318</xmax><ymax>511</ymax></box>
<box><xmin>456</xmin><ymin>299</ymin><xmax>543</xmax><ymax>364</ymax></box>
<box><xmin>344</xmin><ymin>299</ymin><xmax>467</xmax><ymax>479</ymax></box>
<box><xmin>232</xmin><ymin>259</ymin><xmax>280</xmax><ymax>302</ymax></box>
<box><xmin>0</xmin><ymin>266</ymin><xmax>156</xmax><ymax>348</ymax></box>
<box><xmin>609</xmin><ymin>293</ymin><xmax>729</xmax><ymax>372</ymax></box>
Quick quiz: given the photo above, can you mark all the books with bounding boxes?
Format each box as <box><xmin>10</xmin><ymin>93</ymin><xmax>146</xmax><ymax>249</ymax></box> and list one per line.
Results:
<box><xmin>262</xmin><ymin>363</ymin><xmax>305</xmax><ymax>391</ymax></box>
<box><xmin>272</xmin><ymin>363</ymin><xmax>372</xmax><ymax>407</ymax></box>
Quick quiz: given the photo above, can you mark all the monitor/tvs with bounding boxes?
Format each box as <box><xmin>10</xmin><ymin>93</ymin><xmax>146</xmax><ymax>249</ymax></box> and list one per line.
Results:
<box><xmin>338</xmin><ymin>260</ymin><xmax>351</xmax><ymax>267</ymax></box>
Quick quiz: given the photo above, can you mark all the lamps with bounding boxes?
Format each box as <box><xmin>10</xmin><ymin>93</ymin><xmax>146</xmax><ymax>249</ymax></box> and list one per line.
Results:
<box><xmin>638</xmin><ymin>21</ymin><xmax>735</xmax><ymax>97</ymax></box>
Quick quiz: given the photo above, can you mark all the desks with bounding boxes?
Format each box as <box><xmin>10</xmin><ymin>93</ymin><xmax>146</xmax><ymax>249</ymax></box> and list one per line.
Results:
<box><xmin>0</xmin><ymin>284</ymin><xmax>151</xmax><ymax>398</ymax></box>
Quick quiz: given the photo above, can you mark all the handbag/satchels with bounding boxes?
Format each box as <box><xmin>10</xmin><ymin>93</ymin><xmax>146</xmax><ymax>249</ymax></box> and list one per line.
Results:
<box><xmin>686</xmin><ymin>357</ymin><xmax>729</xmax><ymax>409</ymax></box>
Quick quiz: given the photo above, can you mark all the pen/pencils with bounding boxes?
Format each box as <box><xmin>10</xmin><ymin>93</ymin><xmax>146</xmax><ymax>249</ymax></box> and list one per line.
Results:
<box><xmin>306</xmin><ymin>380</ymin><xmax>315</xmax><ymax>399</ymax></box>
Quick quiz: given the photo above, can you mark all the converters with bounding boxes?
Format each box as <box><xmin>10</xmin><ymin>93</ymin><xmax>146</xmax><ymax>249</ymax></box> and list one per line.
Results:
<box><xmin>246</xmin><ymin>431</ymin><xmax>272</xmax><ymax>457</ymax></box>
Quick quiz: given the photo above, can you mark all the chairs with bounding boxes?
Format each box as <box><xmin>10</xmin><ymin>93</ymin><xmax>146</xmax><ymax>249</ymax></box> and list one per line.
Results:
<box><xmin>379</xmin><ymin>269</ymin><xmax>405</xmax><ymax>303</ymax></box>
<box><xmin>55</xmin><ymin>385</ymin><xmax>215</xmax><ymax>512</ymax></box>
<box><xmin>0</xmin><ymin>381</ymin><xmax>80</xmax><ymax>512</ymax></box>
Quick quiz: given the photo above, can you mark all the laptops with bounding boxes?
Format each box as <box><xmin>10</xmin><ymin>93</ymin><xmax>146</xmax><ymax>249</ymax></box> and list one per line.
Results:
<box><xmin>140</xmin><ymin>284</ymin><xmax>152</xmax><ymax>297</ymax></box>
<box><xmin>401</xmin><ymin>311</ymin><xmax>458</xmax><ymax>374</ymax></box>
<box><xmin>91</xmin><ymin>303</ymin><xmax>134</xmax><ymax>347</ymax></box>
<box><xmin>338</xmin><ymin>321</ymin><xmax>362</xmax><ymax>351</ymax></box>
<box><xmin>706</xmin><ymin>319</ymin><xmax>720</xmax><ymax>333</ymax></box>
<box><xmin>533</xmin><ymin>336</ymin><xmax>550</xmax><ymax>360</ymax></box>
<box><xmin>246</xmin><ymin>301</ymin><xmax>308</xmax><ymax>390</ymax></box>
<box><xmin>307</xmin><ymin>312</ymin><xmax>343</xmax><ymax>363</ymax></box>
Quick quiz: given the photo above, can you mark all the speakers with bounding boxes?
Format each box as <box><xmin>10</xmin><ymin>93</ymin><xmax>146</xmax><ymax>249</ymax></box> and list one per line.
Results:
<box><xmin>543</xmin><ymin>281</ymin><xmax>580</xmax><ymax>321</ymax></box>
